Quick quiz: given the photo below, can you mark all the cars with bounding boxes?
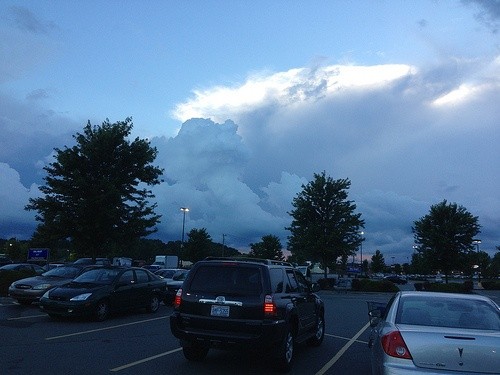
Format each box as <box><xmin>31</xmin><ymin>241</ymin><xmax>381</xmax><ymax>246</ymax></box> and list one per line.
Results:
<box><xmin>40</xmin><ymin>266</ymin><xmax>167</xmax><ymax>322</ymax></box>
<box><xmin>9</xmin><ymin>267</ymin><xmax>81</xmax><ymax>311</ymax></box>
<box><xmin>42</xmin><ymin>255</ymin><xmax>189</xmax><ymax>305</ymax></box>
<box><xmin>383</xmin><ymin>274</ymin><xmax>408</xmax><ymax>285</ymax></box>
<box><xmin>368</xmin><ymin>291</ymin><xmax>500</xmax><ymax>375</ymax></box>
<box><xmin>435</xmin><ymin>277</ymin><xmax>443</xmax><ymax>283</ymax></box>
<box><xmin>0</xmin><ymin>263</ymin><xmax>47</xmax><ymax>297</ymax></box>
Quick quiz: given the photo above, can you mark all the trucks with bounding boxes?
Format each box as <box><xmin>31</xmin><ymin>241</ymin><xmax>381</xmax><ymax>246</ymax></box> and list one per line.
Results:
<box><xmin>296</xmin><ymin>266</ymin><xmax>338</xmax><ymax>292</ymax></box>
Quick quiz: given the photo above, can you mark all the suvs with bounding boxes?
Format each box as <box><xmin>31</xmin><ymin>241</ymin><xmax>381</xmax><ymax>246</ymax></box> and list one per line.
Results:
<box><xmin>170</xmin><ymin>257</ymin><xmax>325</xmax><ymax>372</ymax></box>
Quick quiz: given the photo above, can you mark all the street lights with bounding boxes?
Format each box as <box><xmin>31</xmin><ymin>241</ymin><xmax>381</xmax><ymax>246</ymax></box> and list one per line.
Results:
<box><xmin>357</xmin><ymin>231</ymin><xmax>364</xmax><ymax>263</ymax></box>
<box><xmin>412</xmin><ymin>245</ymin><xmax>418</xmax><ymax>254</ymax></box>
<box><xmin>9</xmin><ymin>243</ymin><xmax>13</xmax><ymax>263</ymax></box>
<box><xmin>180</xmin><ymin>207</ymin><xmax>189</xmax><ymax>242</ymax></box>
<box><xmin>474</xmin><ymin>240</ymin><xmax>482</xmax><ymax>282</ymax></box>
